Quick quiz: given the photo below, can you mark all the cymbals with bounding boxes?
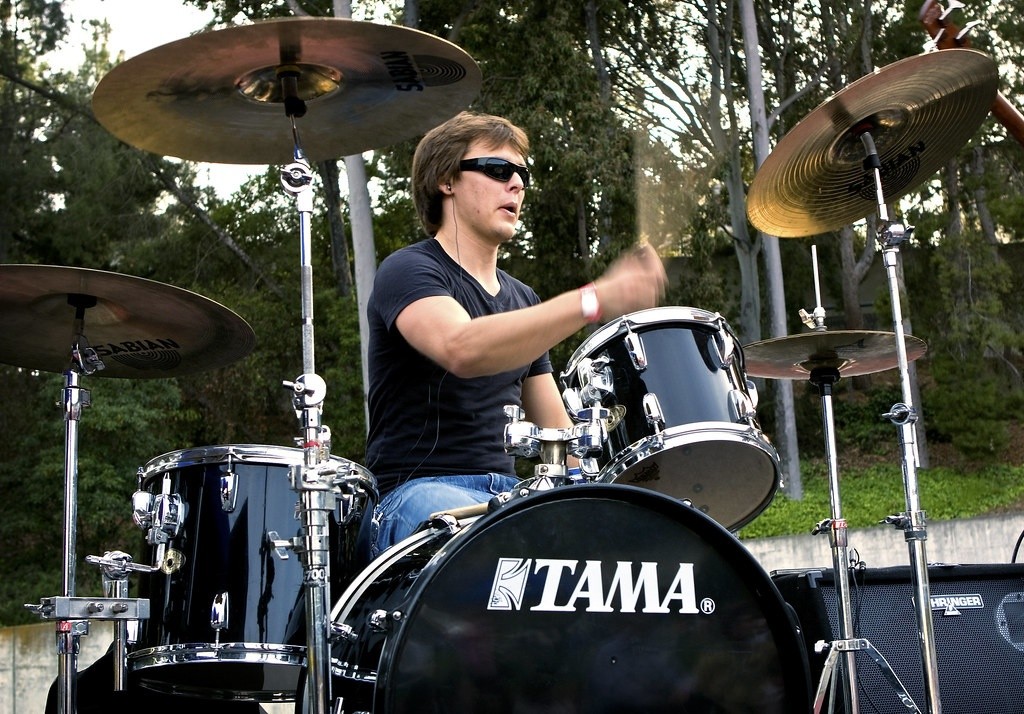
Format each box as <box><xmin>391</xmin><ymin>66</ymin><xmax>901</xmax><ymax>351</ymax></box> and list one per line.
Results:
<box><xmin>741</xmin><ymin>330</ymin><xmax>931</xmax><ymax>378</ymax></box>
<box><xmin>0</xmin><ymin>261</ymin><xmax>258</xmax><ymax>379</ymax></box>
<box><xmin>745</xmin><ymin>47</ymin><xmax>1000</xmax><ymax>239</ymax></box>
<box><xmin>89</xmin><ymin>14</ymin><xmax>484</xmax><ymax>166</ymax></box>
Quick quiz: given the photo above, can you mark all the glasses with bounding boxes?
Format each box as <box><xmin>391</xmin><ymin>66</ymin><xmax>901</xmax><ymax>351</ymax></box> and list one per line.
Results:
<box><xmin>459</xmin><ymin>157</ymin><xmax>530</xmax><ymax>190</ymax></box>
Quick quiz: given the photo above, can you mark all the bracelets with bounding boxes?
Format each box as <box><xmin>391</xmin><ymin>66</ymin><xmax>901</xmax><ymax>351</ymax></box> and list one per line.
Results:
<box><xmin>579</xmin><ymin>281</ymin><xmax>604</xmax><ymax>321</ymax></box>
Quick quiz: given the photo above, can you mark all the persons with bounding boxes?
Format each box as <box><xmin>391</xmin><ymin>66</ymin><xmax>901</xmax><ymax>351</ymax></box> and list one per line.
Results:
<box><xmin>365</xmin><ymin>111</ymin><xmax>666</xmax><ymax>558</ymax></box>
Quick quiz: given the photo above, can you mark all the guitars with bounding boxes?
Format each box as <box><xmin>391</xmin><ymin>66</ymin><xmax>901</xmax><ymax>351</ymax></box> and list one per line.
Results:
<box><xmin>917</xmin><ymin>0</ymin><xmax>1024</xmax><ymax>154</ymax></box>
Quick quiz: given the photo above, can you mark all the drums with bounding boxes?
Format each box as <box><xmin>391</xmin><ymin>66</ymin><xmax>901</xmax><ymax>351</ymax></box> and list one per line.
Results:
<box><xmin>127</xmin><ymin>441</ymin><xmax>379</xmax><ymax>701</ymax></box>
<box><xmin>561</xmin><ymin>304</ymin><xmax>781</xmax><ymax>533</ymax></box>
<box><xmin>298</xmin><ymin>483</ymin><xmax>820</xmax><ymax>713</ymax></box>
